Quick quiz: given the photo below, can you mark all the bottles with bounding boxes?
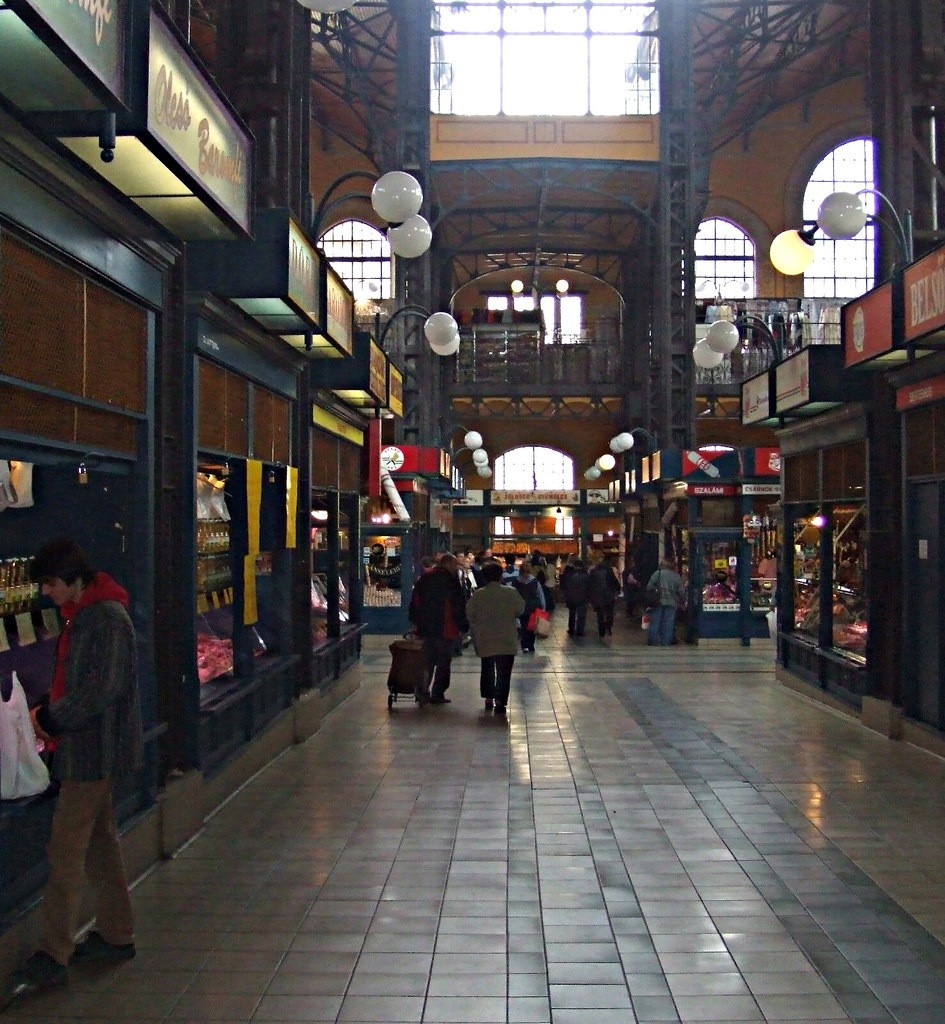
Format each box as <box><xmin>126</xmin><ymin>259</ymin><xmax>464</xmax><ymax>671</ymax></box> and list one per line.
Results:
<box><xmin>196</xmin><ymin>555</ymin><xmax>233</xmax><ymax>596</ymax></box>
<box><xmin>0</xmin><ymin>556</ymin><xmax>40</xmax><ymax>615</ymax></box>
<box><xmin>197</xmin><ymin>517</ymin><xmax>230</xmax><ymax>554</ymax></box>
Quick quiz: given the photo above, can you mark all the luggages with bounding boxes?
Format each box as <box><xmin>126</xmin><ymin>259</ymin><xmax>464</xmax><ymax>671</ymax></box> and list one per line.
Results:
<box><xmin>387</xmin><ymin>633</ymin><xmax>430</xmax><ymax>711</ymax></box>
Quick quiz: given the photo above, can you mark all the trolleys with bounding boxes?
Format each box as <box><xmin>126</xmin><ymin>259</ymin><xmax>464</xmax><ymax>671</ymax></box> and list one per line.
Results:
<box><xmin>388</xmin><ymin>631</ymin><xmax>430</xmax><ymax>710</ymax></box>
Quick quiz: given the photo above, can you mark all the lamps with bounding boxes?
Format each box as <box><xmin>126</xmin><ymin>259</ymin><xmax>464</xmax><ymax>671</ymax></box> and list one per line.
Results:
<box><xmin>439</xmin><ymin>424</ymin><xmax>482</xmax><ymax>451</ymax></box>
<box><xmin>584</xmin><ymin>466</ymin><xmax>620</xmax><ymax>481</ymax></box>
<box><xmin>312</xmin><ymin>170</ymin><xmax>424</xmax><ymax>246</ymax></box>
<box><xmin>317</xmin><ymin>190</ymin><xmax>433</xmax><ymax>259</ymax></box>
<box><xmin>769</xmin><ymin>214</ymin><xmax>904</xmax><ymax>276</ymax></box>
<box><xmin>297</xmin><ymin>1</ymin><xmax>361</xmax><ymax>14</ymax></box>
<box><xmin>555</xmin><ymin>507</ymin><xmax>564</xmax><ymax>520</ymax></box>
<box><xmin>380</xmin><ymin>303</ymin><xmax>458</xmax><ymax>348</ymax></box>
<box><xmin>816</xmin><ymin>188</ymin><xmax>910</xmax><ymax>267</ymax></box>
<box><xmin>452</xmin><ymin>444</ymin><xmax>489</xmax><ymax>467</ymax></box>
<box><xmin>595</xmin><ymin>450</ymin><xmax>637</xmax><ymax>470</ymax></box>
<box><xmin>609</xmin><ymin>427</ymin><xmax>652</xmax><ymax>454</ymax></box>
<box><xmin>706</xmin><ymin>314</ymin><xmax>780</xmax><ymax>363</ymax></box>
<box><xmin>391</xmin><ymin>303</ymin><xmax>461</xmax><ymax>355</ymax></box>
<box><xmin>511</xmin><ymin>279</ymin><xmax>569</xmax><ymax>310</ymax></box>
<box><xmin>459</xmin><ymin>460</ymin><xmax>492</xmax><ymax>479</ymax></box>
<box><xmin>693</xmin><ymin>323</ymin><xmax>776</xmax><ymax>369</ymax></box>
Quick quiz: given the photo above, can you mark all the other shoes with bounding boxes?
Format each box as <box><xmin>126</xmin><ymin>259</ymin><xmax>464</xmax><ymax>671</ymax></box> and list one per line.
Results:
<box><xmin>520</xmin><ymin>641</ymin><xmax>535</xmax><ymax>653</ymax></box>
<box><xmin>485</xmin><ymin>696</ymin><xmax>494</xmax><ymax>710</ymax></box>
<box><xmin>607</xmin><ymin>629</ymin><xmax>612</xmax><ymax>636</ymax></box>
<box><xmin>495</xmin><ymin>700</ymin><xmax>506</xmax><ymax>713</ymax></box>
<box><xmin>599</xmin><ymin>630</ymin><xmax>606</xmax><ymax>637</ymax></box>
<box><xmin>71</xmin><ymin>931</ymin><xmax>137</xmax><ymax>966</ymax></box>
<box><xmin>430</xmin><ymin>695</ymin><xmax>451</xmax><ymax>705</ymax></box>
<box><xmin>567</xmin><ymin>630</ymin><xmax>575</xmax><ymax>634</ymax></box>
<box><xmin>577</xmin><ymin>629</ymin><xmax>584</xmax><ymax>634</ymax></box>
<box><xmin>10</xmin><ymin>950</ymin><xmax>70</xmax><ymax>1000</ymax></box>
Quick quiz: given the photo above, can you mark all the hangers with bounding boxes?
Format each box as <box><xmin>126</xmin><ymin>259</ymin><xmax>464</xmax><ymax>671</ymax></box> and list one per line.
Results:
<box><xmin>704</xmin><ymin>301</ymin><xmax>848</xmax><ymax>324</ymax></box>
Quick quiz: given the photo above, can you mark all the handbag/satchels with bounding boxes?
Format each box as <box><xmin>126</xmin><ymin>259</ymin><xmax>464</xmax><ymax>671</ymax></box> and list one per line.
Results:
<box><xmin>641</xmin><ymin>607</ymin><xmax>652</xmax><ymax>630</ymax></box>
<box><xmin>0</xmin><ymin>672</ymin><xmax>50</xmax><ymax>800</ymax></box>
<box><xmin>642</xmin><ymin>586</ymin><xmax>661</xmax><ymax>607</ymax></box>
<box><xmin>527</xmin><ymin>608</ymin><xmax>551</xmax><ymax>638</ymax></box>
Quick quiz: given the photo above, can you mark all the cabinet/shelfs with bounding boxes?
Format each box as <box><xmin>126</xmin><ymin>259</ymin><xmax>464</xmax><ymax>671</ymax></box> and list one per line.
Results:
<box><xmin>792</xmin><ymin>504</ymin><xmax>867</xmax><ymax>665</ymax></box>
<box><xmin>712</xmin><ymin>309</ymin><xmax>808</xmax><ymax>384</ymax></box>
<box><xmin>0</xmin><ymin>458</ymin><xmax>133</xmax><ymax>815</ymax></box>
<box><xmin>457</xmin><ymin>324</ymin><xmax>544</xmax><ymax>384</ymax></box>
<box><xmin>543</xmin><ymin>315</ymin><xmax>623</xmax><ymax>384</ymax></box>
<box><xmin>197</xmin><ymin>467</ymin><xmax>278</xmax><ymax>699</ymax></box>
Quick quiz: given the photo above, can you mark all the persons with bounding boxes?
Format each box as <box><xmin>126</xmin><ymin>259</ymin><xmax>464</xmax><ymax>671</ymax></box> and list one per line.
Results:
<box><xmin>757</xmin><ymin>549</ymin><xmax>778</xmax><ymax>612</ymax></box>
<box><xmin>418</xmin><ymin>547</ymin><xmax>687</xmax><ymax>653</ymax></box>
<box><xmin>407</xmin><ymin>554</ymin><xmax>469</xmax><ymax>706</ymax></box>
<box><xmin>17</xmin><ymin>536</ymin><xmax>145</xmax><ymax>989</ymax></box>
<box><xmin>464</xmin><ymin>563</ymin><xmax>526</xmax><ymax>720</ymax></box>
